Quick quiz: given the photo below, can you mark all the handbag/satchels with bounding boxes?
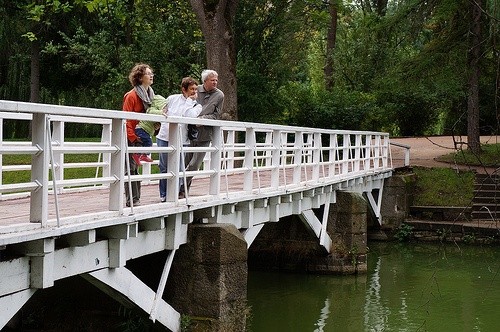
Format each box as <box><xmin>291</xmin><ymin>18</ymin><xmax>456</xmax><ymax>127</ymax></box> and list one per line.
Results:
<box><xmin>187</xmin><ymin>102</ymin><xmax>200</xmax><ymax>141</ymax></box>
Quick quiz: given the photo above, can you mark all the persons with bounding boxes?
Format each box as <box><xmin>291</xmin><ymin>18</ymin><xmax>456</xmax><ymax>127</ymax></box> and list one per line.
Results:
<box><xmin>121</xmin><ymin>64</ymin><xmax>169</xmax><ymax>208</ymax></box>
<box><xmin>153</xmin><ymin>77</ymin><xmax>204</xmax><ymax>203</ymax></box>
<box><xmin>176</xmin><ymin>70</ymin><xmax>224</xmax><ymax>201</ymax></box>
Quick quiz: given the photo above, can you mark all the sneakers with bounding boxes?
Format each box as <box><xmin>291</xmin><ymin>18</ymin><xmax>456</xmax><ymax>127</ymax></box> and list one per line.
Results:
<box><xmin>132</xmin><ymin>152</ymin><xmax>143</xmax><ymax>166</ymax></box>
<box><xmin>139</xmin><ymin>154</ymin><xmax>156</xmax><ymax>164</ymax></box>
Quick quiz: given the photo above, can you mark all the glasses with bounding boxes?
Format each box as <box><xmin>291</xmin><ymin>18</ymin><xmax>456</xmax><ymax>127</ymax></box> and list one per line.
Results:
<box><xmin>143</xmin><ymin>72</ymin><xmax>155</xmax><ymax>77</ymax></box>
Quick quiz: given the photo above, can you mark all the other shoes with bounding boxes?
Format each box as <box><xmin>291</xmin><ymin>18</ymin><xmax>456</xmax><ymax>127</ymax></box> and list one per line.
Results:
<box><xmin>128</xmin><ymin>200</ymin><xmax>141</xmax><ymax>207</ymax></box>
<box><xmin>160</xmin><ymin>194</ymin><xmax>168</xmax><ymax>202</ymax></box>
<box><xmin>179</xmin><ymin>189</ymin><xmax>187</xmax><ymax>199</ymax></box>
